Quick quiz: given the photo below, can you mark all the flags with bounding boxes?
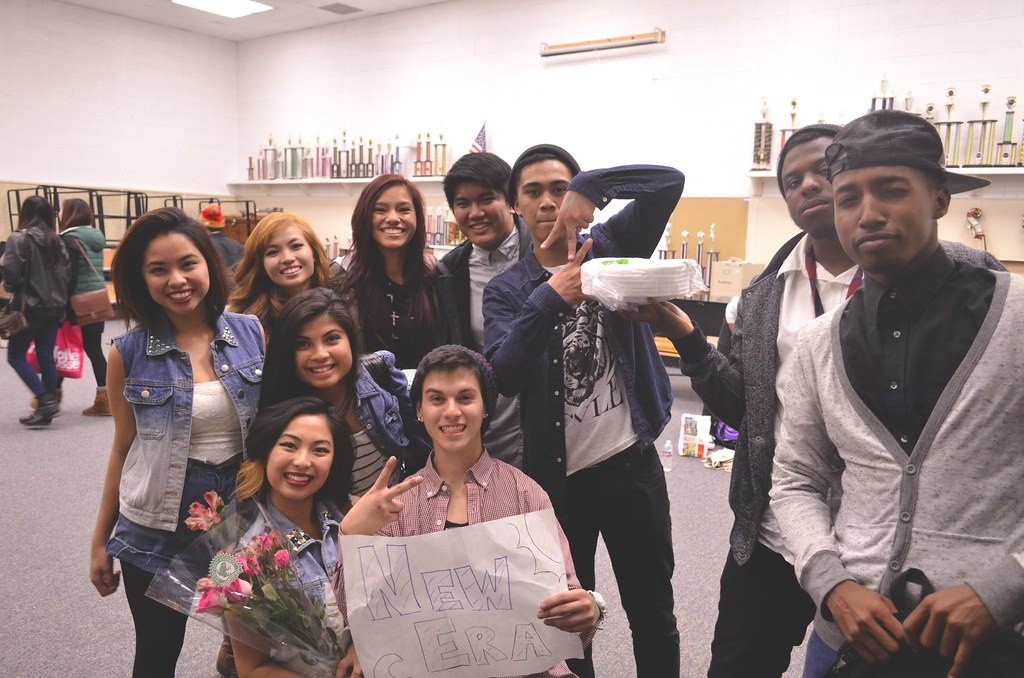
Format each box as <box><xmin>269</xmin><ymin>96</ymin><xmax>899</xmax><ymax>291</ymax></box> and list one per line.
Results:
<box><xmin>469</xmin><ymin>123</ymin><xmax>486</xmax><ymax>154</ymax></box>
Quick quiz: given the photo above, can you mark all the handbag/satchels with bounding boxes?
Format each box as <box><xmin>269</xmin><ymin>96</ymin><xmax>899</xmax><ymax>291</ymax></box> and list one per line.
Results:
<box><xmin>69</xmin><ymin>286</ymin><xmax>115</xmax><ymax>325</ymax></box>
<box><xmin>25</xmin><ymin>321</ymin><xmax>83</xmax><ymax>379</ymax></box>
<box><xmin>0</xmin><ymin>314</ymin><xmax>28</xmax><ymax>340</ymax></box>
<box><xmin>822</xmin><ymin>566</ymin><xmax>1023</xmax><ymax>678</ymax></box>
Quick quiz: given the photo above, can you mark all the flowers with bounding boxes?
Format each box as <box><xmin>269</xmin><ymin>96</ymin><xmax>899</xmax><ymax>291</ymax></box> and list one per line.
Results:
<box><xmin>183</xmin><ymin>488</ymin><xmax>345</xmax><ymax>678</ymax></box>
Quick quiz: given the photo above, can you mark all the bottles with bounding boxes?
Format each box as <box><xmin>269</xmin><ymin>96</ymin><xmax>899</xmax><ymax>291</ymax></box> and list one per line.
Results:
<box><xmin>682</xmin><ymin>417</ymin><xmax>697</xmax><ymax>457</ymax></box>
<box><xmin>661</xmin><ymin>439</ymin><xmax>674</xmax><ymax>472</ymax></box>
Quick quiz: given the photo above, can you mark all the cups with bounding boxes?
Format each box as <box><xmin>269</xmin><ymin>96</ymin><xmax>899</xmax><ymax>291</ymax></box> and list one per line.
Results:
<box><xmin>698</xmin><ymin>444</ymin><xmax>703</xmax><ymax>457</ymax></box>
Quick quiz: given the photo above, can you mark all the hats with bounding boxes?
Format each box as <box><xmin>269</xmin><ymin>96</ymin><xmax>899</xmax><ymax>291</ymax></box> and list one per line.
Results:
<box><xmin>778</xmin><ymin>124</ymin><xmax>842</xmax><ymax>199</ymax></box>
<box><xmin>824</xmin><ymin>109</ymin><xmax>992</xmax><ymax>194</ymax></box>
<box><xmin>200</xmin><ymin>204</ymin><xmax>226</xmax><ymax>230</ymax></box>
<box><xmin>506</xmin><ymin>143</ymin><xmax>581</xmax><ymax>213</ymax></box>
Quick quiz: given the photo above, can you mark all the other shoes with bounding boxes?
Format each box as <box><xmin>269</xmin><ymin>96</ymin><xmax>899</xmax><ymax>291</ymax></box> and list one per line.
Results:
<box><xmin>18</xmin><ymin>402</ymin><xmax>60</xmax><ymax>426</ymax></box>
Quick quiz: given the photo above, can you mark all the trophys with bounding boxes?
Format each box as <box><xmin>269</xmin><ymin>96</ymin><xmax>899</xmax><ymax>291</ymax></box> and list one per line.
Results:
<box><xmin>749</xmin><ymin>78</ymin><xmax>1024</xmax><ymax>171</ymax></box>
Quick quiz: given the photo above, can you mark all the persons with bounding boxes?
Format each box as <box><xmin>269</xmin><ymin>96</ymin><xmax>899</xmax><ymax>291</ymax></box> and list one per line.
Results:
<box><xmin>330</xmin><ymin>345</ymin><xmax>606</xmax><ymax>678</ymax></box>
<box><xmin>440</xmin><ymin>150</ymin><xmax>533</xmax><ymax>355</ymax></box>
<box><xmin>229</xmin><ymin>211</ymin><xmax>330</xmax><ymax>343</ymax></box>
<box><xmin>90</xmin><ymin>206</ymin><xmax>266</xmax><ymax>678</ymax></box>
<box><xmin>268</xmin><ymin>287</ymin><xmax>434</xmax><ymax>499</ymax></box>
<box><xmin>223</xmin><ymin>395</ymin><xmax>361</xmax><ymax>678</ymax></box>
<box><xmin>617</xmin><ymin>124</ymin><xmax>1010</xmax><ymax>678</ymax></box>
<box><xmin>3</xmin><ymin>195</ymin><xmax>112</xmax><ymax>426</ymax></box>
<box><xmin>199</xmin><ymin>202</ymin><xmax>245</xmax><ymax>270</ymax></box>
<box><xmin>331</xmin><ymin>174</ymin><xmax>463</xmax><ymax>390</ymax></box>
<box><xmin>482</xmin><ymin>144</ymin><xmax>685</xmax><ymax>678</ymax></box>
<box><xmin>767</xmin><ymin>109</ymin><xmax>1024</xmax><ymax>678</ymax></box>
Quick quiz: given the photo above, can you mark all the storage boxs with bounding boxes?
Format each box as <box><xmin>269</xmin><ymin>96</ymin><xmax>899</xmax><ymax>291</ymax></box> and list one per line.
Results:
<box><xmin>709</xmin><ymin>260</ymin><xmax>765</xmax><ymax>303</ymax></box>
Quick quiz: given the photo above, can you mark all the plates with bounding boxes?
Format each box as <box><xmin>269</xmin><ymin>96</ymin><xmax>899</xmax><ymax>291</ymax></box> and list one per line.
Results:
<box><xmin>581</xmin><ymin>256</ymin><xmax>690</xmax><ymax>304</ymax></box>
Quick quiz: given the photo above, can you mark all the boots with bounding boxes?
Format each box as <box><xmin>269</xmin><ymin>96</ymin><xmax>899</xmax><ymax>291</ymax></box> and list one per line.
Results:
<box><xmin>83</xmin><ymin>387</ymin><xmax>112</xmax><ymax>416</ymax></box>
<box><xmin>30</xmin><ymin>388</ymin><xmax>62</xmax><ymax>409</ymax></box>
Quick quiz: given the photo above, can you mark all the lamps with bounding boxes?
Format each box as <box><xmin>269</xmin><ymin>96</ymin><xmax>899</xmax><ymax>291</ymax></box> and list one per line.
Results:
<box><xmin>537</xmin><ymin>27</ymin><xmax>672</xmax><ymax>65</ymax></box>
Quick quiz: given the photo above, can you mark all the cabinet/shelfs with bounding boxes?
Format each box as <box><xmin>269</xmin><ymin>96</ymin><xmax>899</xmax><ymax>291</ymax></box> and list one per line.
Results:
<box><xmin>226</xmin><ymin>175</ymin><xmax>457</xmax><ymax>250</ymax></box>
